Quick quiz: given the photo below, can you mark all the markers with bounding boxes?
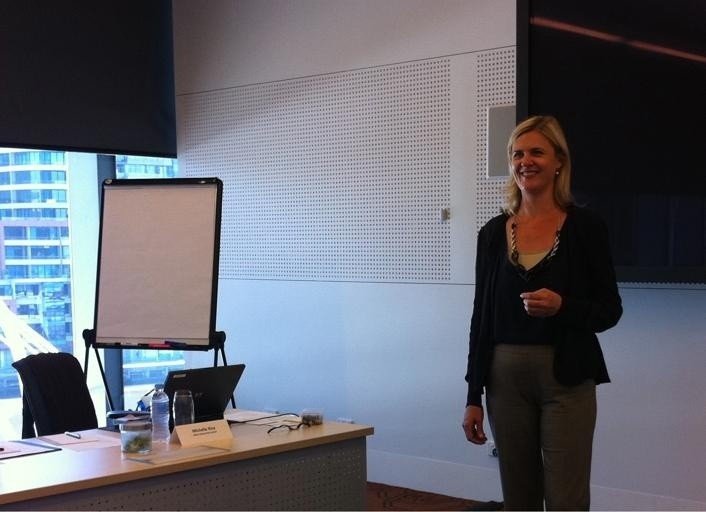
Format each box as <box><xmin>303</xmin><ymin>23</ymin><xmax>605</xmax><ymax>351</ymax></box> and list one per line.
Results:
<box><xmin>139</xmin><ymin>344</ymin><xmax>174</xmax><ymax>350</ymax></box>
<box><xmin>116</xmin><ymin>342</ymin><xmax>143</xmax><ymax>348</ymax></box>
<box><xmin>164</xmin><ymin>341</ymin><xmax>188</xmax><ymax>347</ymax></box>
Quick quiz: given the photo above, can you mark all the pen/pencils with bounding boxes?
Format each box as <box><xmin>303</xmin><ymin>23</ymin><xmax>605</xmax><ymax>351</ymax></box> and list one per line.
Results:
<box><xmin>64</xmin><ymin>431</ymin><xmax>81</xmax><ymax>438</ymax></box>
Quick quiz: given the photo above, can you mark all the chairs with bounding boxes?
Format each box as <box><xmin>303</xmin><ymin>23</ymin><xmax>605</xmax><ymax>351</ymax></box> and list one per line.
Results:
<box><xmin>9</xmin><ymin>351</ymin><xmax>98</xmax><ymax>436</ymax></box>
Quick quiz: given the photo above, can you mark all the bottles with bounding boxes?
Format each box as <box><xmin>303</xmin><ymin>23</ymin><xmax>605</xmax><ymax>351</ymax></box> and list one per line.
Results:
<box><xmin>119</xmin><ymin>422</ymin><xmax>152</xmax><ymax>452</ymax></box>
<box><xmin>152</xmin><ymin>384</ymin><xmax>171</xmax><ymax>441</ymax></box>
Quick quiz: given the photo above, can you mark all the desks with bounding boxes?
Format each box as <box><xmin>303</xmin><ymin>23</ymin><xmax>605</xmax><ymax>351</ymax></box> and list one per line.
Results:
<box><xmin>0</xmin><ymin>409</ymin><xmax>374</xmax><ymax>512</ymax></box>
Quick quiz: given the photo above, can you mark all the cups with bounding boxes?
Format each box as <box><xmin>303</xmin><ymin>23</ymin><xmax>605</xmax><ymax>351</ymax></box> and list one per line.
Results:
<box><xmin>172</xmin><ymin>390</ymin><xmax>195</xmax><ymax>425</ymax></box>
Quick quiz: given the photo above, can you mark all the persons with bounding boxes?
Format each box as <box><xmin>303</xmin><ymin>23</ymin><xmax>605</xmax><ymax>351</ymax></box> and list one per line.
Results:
<box><xmin>462</xmin><ymin>113</ymin><xmax>624</xmax><ymax>512</ymax></box>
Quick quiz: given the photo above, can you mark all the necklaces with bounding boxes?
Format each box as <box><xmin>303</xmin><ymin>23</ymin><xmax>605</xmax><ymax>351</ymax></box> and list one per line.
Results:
<box><xmin>509</xmin><ymin>205</ymin><xmax>561</xmax><ymax>271</ymax></box>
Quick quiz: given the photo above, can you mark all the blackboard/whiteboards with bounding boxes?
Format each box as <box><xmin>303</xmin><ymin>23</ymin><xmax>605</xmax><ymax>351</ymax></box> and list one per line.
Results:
<box><xmin>93</xmin><ymin>177</ymin><xmax>223</xmax><ymax>350</ymax></box>
